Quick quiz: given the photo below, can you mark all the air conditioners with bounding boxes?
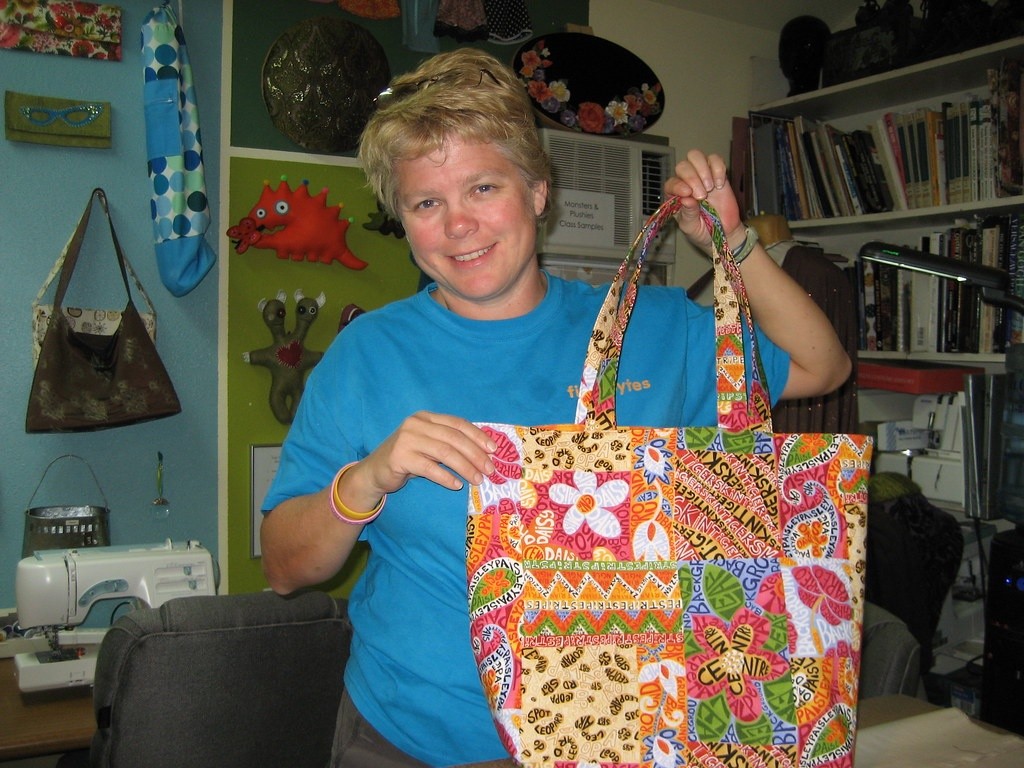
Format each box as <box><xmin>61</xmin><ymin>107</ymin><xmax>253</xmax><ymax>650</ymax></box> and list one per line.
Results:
<box><xmin>536</xmin><ymin>127</ymin><xmax>676</xmax><ymax>270</ymax></box>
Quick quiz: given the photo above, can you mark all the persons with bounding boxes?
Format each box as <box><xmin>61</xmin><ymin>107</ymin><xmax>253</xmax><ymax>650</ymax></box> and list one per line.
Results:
<box><xmin>260</xmin><ymin>48</ymin><xmax>853</xmax><ymax>768</ymax></box>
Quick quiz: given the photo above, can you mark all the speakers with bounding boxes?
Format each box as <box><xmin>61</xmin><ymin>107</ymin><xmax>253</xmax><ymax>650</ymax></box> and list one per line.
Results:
<box><xmin>980</xmin><ymin>528</ymin><xmax>1024</xmax><ymax>738</ymax></box>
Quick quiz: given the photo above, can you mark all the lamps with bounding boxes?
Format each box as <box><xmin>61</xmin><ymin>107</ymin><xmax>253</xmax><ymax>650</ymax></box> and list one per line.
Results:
<box><xmin>858</xmin><ymin>242</ymin><xmax>1024</xmax><ymax>316</ymax></box>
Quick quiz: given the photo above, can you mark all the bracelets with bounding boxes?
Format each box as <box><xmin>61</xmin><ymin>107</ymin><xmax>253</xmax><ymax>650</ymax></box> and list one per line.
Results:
<box><xmin>708</xmin><ymin>223</ymin><xmax>759</xmax><ymax>266</ymax></box>
<box><xmin>329</xmin><ymin>461</ymin><xmax>387</xmax><ymax>525</ymax></box>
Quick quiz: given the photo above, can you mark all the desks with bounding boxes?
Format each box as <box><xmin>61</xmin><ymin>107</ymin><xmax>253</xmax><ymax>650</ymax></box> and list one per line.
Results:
<box><xmin>0</xmin><ymin>643</ymin><xmax>99</xmax><ymax>763</ymax></box>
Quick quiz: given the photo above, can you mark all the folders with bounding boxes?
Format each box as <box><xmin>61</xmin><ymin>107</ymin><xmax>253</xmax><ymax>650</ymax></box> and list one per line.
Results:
<box><xmin>963</xmin><ymin>371</ymin><xmax>982</xmax><ymax>517</ymax></box>
<box><xmin>984</xmin><ymin>373</ymin><xmax>1006</xmax><ymax>521</ymax></box>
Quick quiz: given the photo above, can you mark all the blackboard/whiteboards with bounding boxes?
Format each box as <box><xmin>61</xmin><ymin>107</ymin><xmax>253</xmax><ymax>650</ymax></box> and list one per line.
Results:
<box><xmin>249</xmin><ymin>443</ymin><xmax>367</xmax><ymax>560</ymax></box>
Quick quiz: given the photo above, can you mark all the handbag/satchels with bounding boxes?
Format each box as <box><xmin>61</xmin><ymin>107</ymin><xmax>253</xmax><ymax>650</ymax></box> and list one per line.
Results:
<box><xmin>25</xmin><ymin>187</ymin><xmax>181</xmax><ymax>433</ymax></box>
<box><xmin>34</xmin><ymin>187</ymin><xmax>157</xmax><ymax>373</ymax></box>
<box><xmin>0</xmin><ymin>1</ymin><xmax>124</xmax><ymax>62</ymax></box>
<box><xmin>466</xmin><ymin>196</ymin><xmax>874</xmax><ymax>768</ymax></box>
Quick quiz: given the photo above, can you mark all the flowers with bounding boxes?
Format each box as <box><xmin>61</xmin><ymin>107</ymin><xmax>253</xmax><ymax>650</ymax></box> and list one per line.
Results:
<box><xmin>517</xmin><ymin>39</ymin><xmax>664</xmax><ymax>138</ymax></box>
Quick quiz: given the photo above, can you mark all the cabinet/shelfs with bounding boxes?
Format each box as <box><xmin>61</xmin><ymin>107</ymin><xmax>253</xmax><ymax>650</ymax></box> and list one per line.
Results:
<box><xmin>747</xmin><ymin>35</ymin><xmax>1024</xmax><ymax>526</ymax></box>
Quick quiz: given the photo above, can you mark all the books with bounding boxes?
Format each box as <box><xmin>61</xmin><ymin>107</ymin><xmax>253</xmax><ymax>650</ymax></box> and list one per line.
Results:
<box><xmin>744</xmin><ymin>58</ymin><xmax>1024</xmax><ymax>354</ymax></box>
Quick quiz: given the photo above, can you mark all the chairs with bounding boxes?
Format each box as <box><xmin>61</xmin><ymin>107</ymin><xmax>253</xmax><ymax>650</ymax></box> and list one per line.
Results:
<box><xmin>56</xmin><ymin>589</ymin><xmax>354</xmax><ymax>768</ymax></box>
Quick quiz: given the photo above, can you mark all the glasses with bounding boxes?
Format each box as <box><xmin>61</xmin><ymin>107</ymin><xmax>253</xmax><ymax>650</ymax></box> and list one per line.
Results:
<box><xmin>371</xmin><ymin>67</ymin><xmax>502</xmax><ymax>109</ymax></box>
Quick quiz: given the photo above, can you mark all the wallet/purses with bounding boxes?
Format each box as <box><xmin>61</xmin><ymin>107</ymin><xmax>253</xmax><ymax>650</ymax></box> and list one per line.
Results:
<box><xmin>4</xmin><ymin>91</ymin><xmax>111</xmax><ymax>149</ymax></box>
